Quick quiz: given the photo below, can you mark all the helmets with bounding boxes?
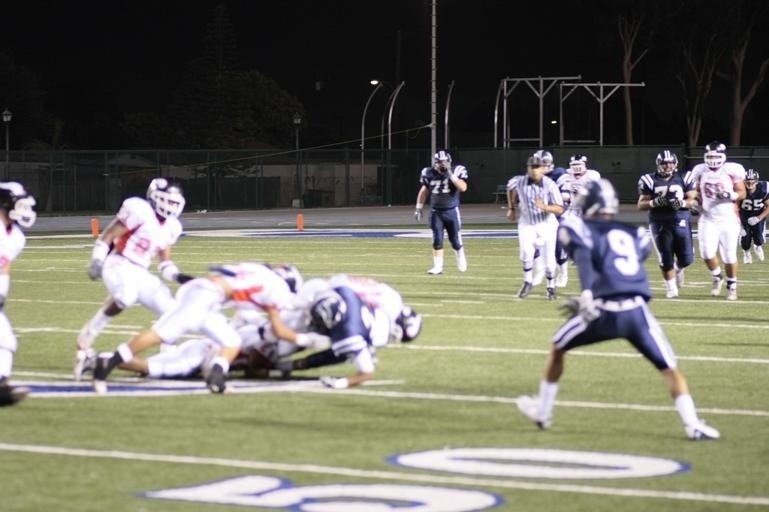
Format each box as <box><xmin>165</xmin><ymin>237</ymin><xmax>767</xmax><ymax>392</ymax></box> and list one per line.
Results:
<box><xmin>266</xmin><ymin>263</ymin><xmax>302</xmax><ymax>295</ymax></box>
<box><xmin>656</xmin><ymin>151</ymin><xmax>678</xmax><ymax>176</ymax></box>
<box><xmin>297</xmin><ymin>278</ymin><xmax>347</xmax><ymax>326</ymax></box>
<box><xmin>0</xmin><ymin>182</ymin><xmax>38</xmax><ymax>228</ymax></box>
<box><xmin>147</xmin><ymin>179</ymin><xmax>186</xmax><ymax>223</ymax></box>
<box><xmin>744</xmin><ymin>168</ymin><xmax>760</xmax><ymax>190</ymax></box>
<box><xmin>573</xmin><ymin>177</ymin><xmax>620</xmax><ymax>221</ymax></box>
<box><xmin>432</xmin><ymin>149</ymin><xmax>453</xmax><ymax>176</ymax></box>
<box><xmin>702</xmin><ymin>141</ymin><xmax>726</xmax><ymax>169</ymax></box>
<box><xmin>392</xmin><ymin>307</ymin><xmax>422</xmax><ymax>344</ymax></box>
<box><xmin>526</xmin><ymin>149</ymin><xmax>590</xmax><ymax>176</ymax></box>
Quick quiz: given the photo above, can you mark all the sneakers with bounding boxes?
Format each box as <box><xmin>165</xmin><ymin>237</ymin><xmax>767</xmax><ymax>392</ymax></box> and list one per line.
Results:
<box><xmin>206</xmin><ymin>358</ymin><xmax>227</xmax><ymax>397</ymax></box>
<box><xmin>75</xmin><ymin>322</ymin><xmax>135</xmax><ymax>394</ymax></box>
<box><xmin>518</xmin><ymin>268</ymin><xmax>569</xmax><ymax>301</ymax></box>
<box><xmin>0</xmin><ymin>378</ymin><xmax>29</xmax><ymax>408</ymax></box>
<box><xmin>455</xmin><ymin>260</ymin><xmax>467</xmax><ymax>272</ymax></box>
<box><xmin>661</xmin><ymin>246</ymin><xmax>763</xmax><ymax>301</ymax></box>
<box><xmin>427</xmin><ymin>267</ymin><xmax>442</xmax><ymax>276</ymax></box>
<box><xmin>517</xmin><ymin>394</ymin><xmax>553</xmax><ymax>430</ymax></box>
<box><xmin>686</xmin><ymin>421</ymin><xmax>720</xmax><ymax>440</ymax></box>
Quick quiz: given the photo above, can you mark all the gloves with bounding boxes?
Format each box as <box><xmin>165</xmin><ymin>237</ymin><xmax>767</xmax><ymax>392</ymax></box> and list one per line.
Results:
<box><xmin>85</xmin><ymin>258</ymin><xmax>104</xmax><ymax>282</ymax></box>
<box><xmin>439</xmin><ymin>160</ymin><xmax>450</xmax><ymax>172</ymax></box>
<box><xmin>651</xmin><ymin>194</ymin><xmax>670</xmax><ymax>207</ymax></box>
<box><xmin>670</xmin><ymin>198</ymin><xmax>683</xmax><ymax>209</ymax></box>
<box><xmin>297</xmin><ymin>334</ymin><xmax>329</xmax><ymax>350</ymax></box>
<box><xmin>161</xmin><ymin>261</ymin><xmax>192</xmax><ymax>281</ymax></box>
<box><xmin>318</xmin><ymin>375</ymin><xmax>347</xmax><ymax>388</ymax></box>
<box><xmin>747</xmin><ymin>217</ymin><xmax>760</xmax><ymax>227</ymax></box>
<box><xmin>717</xmin><ymin>190</ymin><xmax>730</xmax><ymax>199</ymax></box>
<box><xmin>555</xmin><ymin>296</ymin><xmax>581</xmax><ymax>320</ymax></box>
<box><xmin>412</xmin><ymin>205</ymin><xmax>424</xmax><ymax>222</ymax></box>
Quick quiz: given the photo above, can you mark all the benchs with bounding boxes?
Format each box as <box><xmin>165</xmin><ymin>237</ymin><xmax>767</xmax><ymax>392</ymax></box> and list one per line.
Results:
<box><xmin>490</xmin><ymin>183</ymin><xmax>510</xmax><ymax>206</ymax></box>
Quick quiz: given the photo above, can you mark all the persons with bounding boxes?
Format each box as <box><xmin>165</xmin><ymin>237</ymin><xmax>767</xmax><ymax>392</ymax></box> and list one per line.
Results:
<box><xmin>691</xmin><ymin>143</ymin><xmax>747</xmax><ymax>299</ymax></box>
<box><xmin>638</xmin><ymin>150</ymin><xmax>697</xmax><ymax>297</ymax></box>
<box><xmin>75</xmin><ymin>178</ymin><xmax>196</xmax><ymax>352</ymax></box>
<box><xmin>509</xmin><ymin>150</ymin><xmax>600</xmax><ymax>299</ymax></box>
<box><xmin>75</xmin><ymin>264</ymin><xmax>420</xmax><ymax>392</ymax></box>
<box><xmin>516</xmin><ymin>179</ymin><xmax>722</xmax><ymax>441</ymax></box>
<box><xmin>735</xmin><ymin>169</ymin><xmax>769</xmax><ymax>263</ymax></box>
<box><xmin>414</xmin><ymin>150</ymin><xmax>468</xmax><ymax>275</ymax></box>
<box><xmin>0</xmin><ymin>182</ymin><xmax>38</xmax><ymax>406</ymax></box>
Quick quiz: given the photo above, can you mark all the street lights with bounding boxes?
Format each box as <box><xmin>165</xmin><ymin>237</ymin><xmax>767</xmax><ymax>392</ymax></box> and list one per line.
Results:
<box><xmin>291</xmin><ymin>110</ymin><xmax>306</xmax><ymax>207</ymax></box>
<box><xmin>369</xmin><ymin>78</ymin><xmax>396</xmax><ymax>95</ymax></box>
<box><xmin>2</xmin><ymin>107</ymin><xmax>15</xmax><ymax>181</ymax></box>
